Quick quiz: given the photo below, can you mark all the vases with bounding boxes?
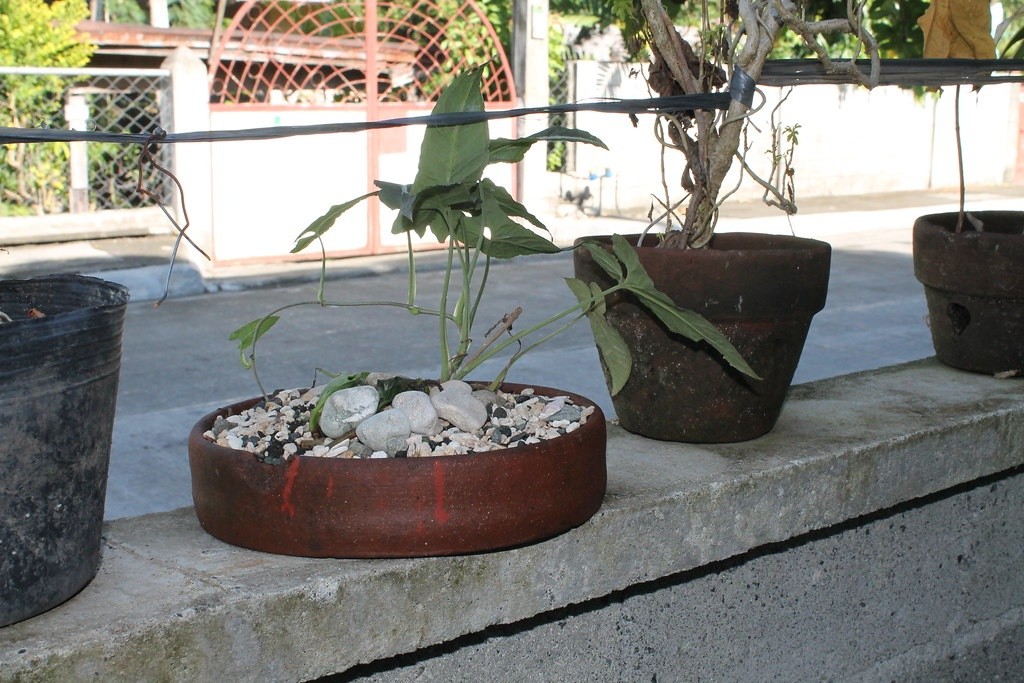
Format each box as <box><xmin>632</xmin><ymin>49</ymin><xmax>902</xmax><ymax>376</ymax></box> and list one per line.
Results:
<box><xmin>0</xmin><ymin>271</ymin><xmax>132</xmax><ymax>621</ymax></box>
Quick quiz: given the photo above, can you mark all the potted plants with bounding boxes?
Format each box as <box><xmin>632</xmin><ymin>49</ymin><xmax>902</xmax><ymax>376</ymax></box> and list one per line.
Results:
<box><xmin>184</xmin><ymin>55</ymin><xmax>755</xmax><ymax>559</ymax></box>
<box><xmin>912</xmin><ymin>82</ymin><xmax>1024</xmax><ymax>379</ymax></box>
<box><xmin>568</xmin><ymin>0</ymin><xmax>887</xmax><ymax>445</ymax></box>
